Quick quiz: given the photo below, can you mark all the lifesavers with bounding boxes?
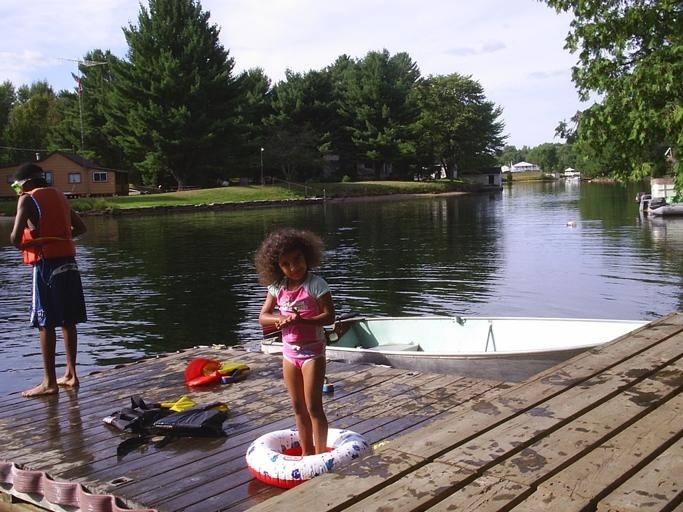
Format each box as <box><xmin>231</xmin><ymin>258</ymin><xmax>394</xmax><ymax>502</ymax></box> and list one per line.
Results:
<box><xmin>246</xmin><ymin>427</ymin><xmax>372</xmax><ymax>489</ymax></box>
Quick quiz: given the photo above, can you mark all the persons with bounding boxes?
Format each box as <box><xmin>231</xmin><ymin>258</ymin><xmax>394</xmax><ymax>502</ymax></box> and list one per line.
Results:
<box><xmin>255</xmin><ymin>225</ymin><xmax>336</xmax><ymax>458</ymax></box>
<box><xmin>10</xmin><ymin>164</ymin><xmax>88</xmax><ymax>397</ymax></box>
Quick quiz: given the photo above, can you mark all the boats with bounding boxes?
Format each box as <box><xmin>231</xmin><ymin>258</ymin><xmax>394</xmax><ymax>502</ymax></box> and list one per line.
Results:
<box><xmin>260</xmin><ymin>313</ymin><xmax>649</xmax><ymax>384</ymax></box>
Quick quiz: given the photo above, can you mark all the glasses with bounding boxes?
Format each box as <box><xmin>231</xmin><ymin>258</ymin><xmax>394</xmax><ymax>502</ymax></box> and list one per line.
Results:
<box><xmin>10</xmin><ymin>180</ymin><xmax>25</xmax><ymax>196</ymax></box>
